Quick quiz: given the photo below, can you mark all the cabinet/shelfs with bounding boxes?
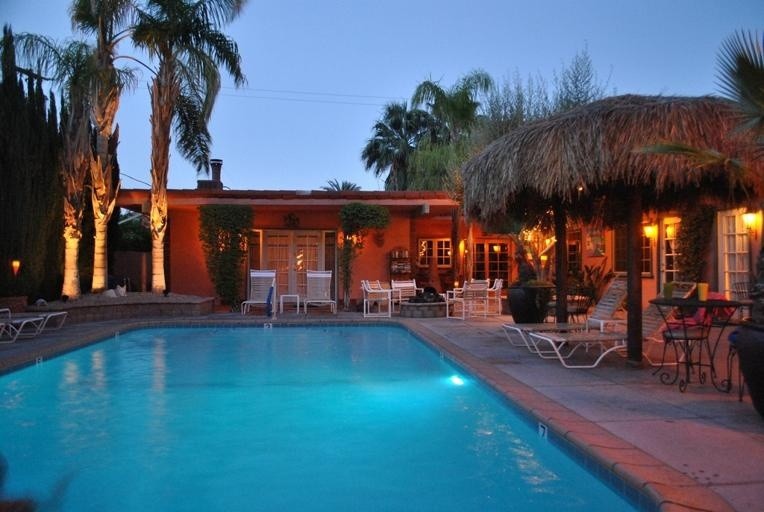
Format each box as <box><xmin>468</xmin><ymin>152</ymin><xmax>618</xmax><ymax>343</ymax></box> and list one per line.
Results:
<box><xmin>390</xmin><ymin>257</ymin><xmax>412</xmax><ymax>274</ymax></box>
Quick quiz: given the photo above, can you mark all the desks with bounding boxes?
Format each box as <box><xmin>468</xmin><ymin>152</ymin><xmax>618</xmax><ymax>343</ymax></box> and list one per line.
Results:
<box><xmin>649</xmin><ymin>295</ymin><xmax>754</xmax><ymax>392</ymax></box>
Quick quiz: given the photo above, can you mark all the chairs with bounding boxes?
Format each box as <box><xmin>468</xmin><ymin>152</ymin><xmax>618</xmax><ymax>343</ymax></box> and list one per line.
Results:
<box><xmin>446</xmin><ymin>279</ymin><xmax>504</xmax><ymax>321</ymax></box>
<box><xmin>240</xmin><ymin>269</ymin><xmax>278</xmax><ymax>319</ymax></box>
<box><xmin>391</xmin><ymin>279</ymin><xmax>425</xmax><ymax>313</ymax></box>
<box><xmin>361</xmin><ymin>280</ymin><xmax>402</xmax><ymax>318</ymax></box>
<box><xmin>0</xmin><ymin>308</ymin><xmax>43</xmax><ymax>344</ymax></box>
<box><xmin>652</xmin><ymin>306</ymin><xmax>717</xmax><ymax>377</ymax></box>
<box><xmin>304</xmin><ymin>269</ymin><xmax>338</xmax><ymax>315</ymax></box>
<box><xmin>546</xmin><ymin>283</ymin><xmax>595</xmax><ymax>333</ymax></box>
<box><xmin>725</xmin><ymin>329</ymin><xmax>750</xmax><ymax>402</ymax></box>
<box><xmin>503</xmin><ymin>273</ymin><xmax>629</xmax><ymax>354</ymax></box>
<box><xmin>0</xmin><ymin>299</ymin><xmax>68</xmax><ymax>335</ymax></box>
<box><xmin>527</xmin><ymin>281</ymin><xmax>696</xmax><ymax>369</ymax></box>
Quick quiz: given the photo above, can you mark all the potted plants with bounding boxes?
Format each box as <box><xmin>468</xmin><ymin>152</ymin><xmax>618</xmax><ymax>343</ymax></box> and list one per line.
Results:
<box><xmin>499</xmin><ymin>225</ymin><xmax>558</xmax><ymax>323</ymax></box>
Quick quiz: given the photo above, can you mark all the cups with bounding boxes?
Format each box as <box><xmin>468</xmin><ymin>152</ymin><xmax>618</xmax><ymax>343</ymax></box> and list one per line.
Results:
<box><xmin>664</xmin><ymin>282</ymin><xmax>674</xmax><ymax>300</ymax></box>
<box><xmin>698</xmin><ymin>282</ymin><xmax>709</xmax><ymax>301</ymax></box>
